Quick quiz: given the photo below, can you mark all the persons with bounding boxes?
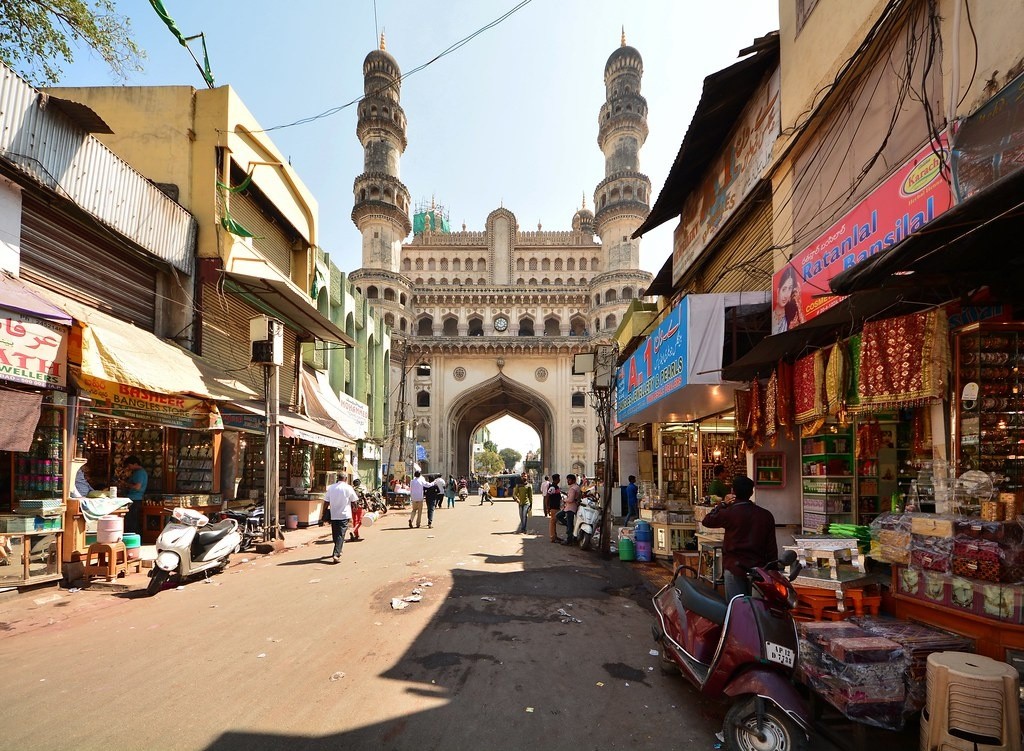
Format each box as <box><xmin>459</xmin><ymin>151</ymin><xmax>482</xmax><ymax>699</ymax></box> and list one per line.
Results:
<box><xmin>772</xmin><ymin>266</ymin><xmax>806</xmax><ymax>337</ymax></box>
<box><xmin>321</xmin><ymin>471</ymin><xmax>359</xmax><ymax>562</ymax></box>
<box><xmin>71</xmin><ymin>469</ymin><xmax>95</xmax><ymax>498</ymax></box>
<box><xmin>623</xmin><ymin>475</ymin><xmax>638</xmax><ymax>527</ymax></box>
<box><xmin>512</xmin><ymin>472</ymin><xmax>533</xmax><ymax>534</ymax></box>
<box><xmin>119</xmin><ymin>457</ymin><xmax>148</xmax><ymax>532</ymax></box>
<box><xmin>540</xmin><ymin>472</ymin><xmax>590</xmax><ymax>546</ymax></box>
<box><xmin>383</xmin><ymin>468</ymin><xmax>518</xmax><ymax>507</ymax></box>
<box><xmin>409</xmin><ymin>471</ymin><xmax>441</xmax><ymax>528</ymax></box>
<box><xmin>701</xmin><ymin>473</ymin><xmax>779</xmax><ymax>601</ymax></box>
<box><xmin>349</xmin><ymin>479</ymin><xmax>373</xmax><ymax>542</ymax></box>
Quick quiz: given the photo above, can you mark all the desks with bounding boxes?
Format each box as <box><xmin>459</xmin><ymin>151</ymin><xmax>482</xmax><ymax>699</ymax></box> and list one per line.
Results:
<box><xmin>786</xmin><ymin>533</ymin><xmax>1024</xmax><ymax>662</ymax></box>
<box><xmin>0</xmin><ymin>529</ymin><xmax>65</xmax><ymax>580</ymax></box>
<box><xmin>388</xmin><ymin>492</ymin><xmax>407</xmax><ymax>508</ymax></box>
<box><xmin>286</xmin><ymin>500</ymin><xmax>324</xmax><ymax>525</ymax></box>
<box><xmin>140</xmin><ymin>505</ymin><xmax>221</xmax><ymax>543</ymax></box>
<box><xmin>698</xmin><ymin>542</ymin><xmax>725</xmax><ymax>590</ymax></box>
<box><xmin>652</xmin><ymin>523</ymin><xmax>696</xmax><ymax>555</ymax></box>
<box><xmin>63</xmin><ymin>497</ymin><xmax>129</xmax><ymax>562</ymax></box>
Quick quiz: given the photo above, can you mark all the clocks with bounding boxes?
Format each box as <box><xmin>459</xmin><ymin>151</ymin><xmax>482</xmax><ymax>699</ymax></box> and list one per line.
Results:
<box><xmin>494</xmin><ymin>317</ymin><xmax>508</xmax><ymax>331</ymax></box>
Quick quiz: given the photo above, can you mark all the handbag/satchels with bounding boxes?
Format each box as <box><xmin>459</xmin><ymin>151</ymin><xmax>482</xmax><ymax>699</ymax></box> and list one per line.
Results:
<box><xmin>451</xmin><ymin>479</ymin><xmax>457</xmax><ymax>492</ymax></box>
<box><xmin>322</xmin><ymin>509</ymin><xmax>331</xmax><ymax>521</ymax></box>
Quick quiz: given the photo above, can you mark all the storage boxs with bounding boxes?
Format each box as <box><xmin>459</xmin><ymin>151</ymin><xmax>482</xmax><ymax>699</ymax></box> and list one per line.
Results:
<box><xmin>910</xmin><ymin>518</ymin><xmax>954</xmax><ymax>537</ymax></box>
<box><xmin>0</xmin><ymin>517</ymin><xmax>61</xmax><ymax>533</ymax></box>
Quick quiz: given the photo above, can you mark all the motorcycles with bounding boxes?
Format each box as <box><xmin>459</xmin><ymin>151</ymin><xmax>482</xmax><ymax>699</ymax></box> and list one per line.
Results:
<box><xmin>218</xmin><ymin>486</ymin><xmax>285</xmax><ymax>552</ymax></box>
<box><xmin>363</xmin><ymin>485</ymin><xmax>388</xmax><ymax>513</ymax></box>
<box><xmin>486</xmin><ymin>473</ymin><xmax>523</xmax><ymax>498</ymax></box>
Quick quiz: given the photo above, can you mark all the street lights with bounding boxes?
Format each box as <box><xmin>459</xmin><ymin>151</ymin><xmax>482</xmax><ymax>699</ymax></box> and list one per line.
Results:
<box><xmin>398</xmin><ymin>363</ymin><xmax>431</xmax><ymax>463</ymax></box>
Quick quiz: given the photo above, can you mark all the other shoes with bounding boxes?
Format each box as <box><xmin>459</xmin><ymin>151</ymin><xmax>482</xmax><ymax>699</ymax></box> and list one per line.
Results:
<box><xmin>333</xmin><ymin>556</ymin><xmax>340</xmax><ymax>563</ymax></box>
<box><xmin>350</xmin><ymin>532</ymin><xmax>358</xmax><ymax>541</ymax></box>
<box><xmin>553</xmin><ymin>539</ymin><xmax>560</xmax><ymax>543</ymax></box>
<box><xmin>559</xmin><ymin>522</ymin><xmax>568</xmax><ymax>525</ymax></box>
<box><xmin>561</xmin><ymin>539</ymin><xmax>571</xmax><ymax>546</ymax></box>
<box><xmin>556</xmin><ymin>537</ymin><xmax>564</xmax><ymax>541</ymax></box>
<box><xmin>417</xmin><ymin>524</ymin><xmax>420</xmax><ymax>528</ymax></box>
<box><xmin>409</xmin><ymin>519</ymin><xmax>413</xmax><ymax>528</ymax></box>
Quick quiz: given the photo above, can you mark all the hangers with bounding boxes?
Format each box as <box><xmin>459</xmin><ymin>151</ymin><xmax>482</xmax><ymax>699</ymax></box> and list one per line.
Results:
<box><xmin>737</xmin><ymin>293</ymin><xmax>940</xmax><ymax>392</ymax></box>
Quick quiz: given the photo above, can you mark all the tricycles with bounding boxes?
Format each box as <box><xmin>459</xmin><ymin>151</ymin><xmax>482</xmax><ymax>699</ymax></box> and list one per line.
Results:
<box><xmin>387</xmin><ymin>490</ymin><xmax>411</xmax><ymax>509</ymax></box>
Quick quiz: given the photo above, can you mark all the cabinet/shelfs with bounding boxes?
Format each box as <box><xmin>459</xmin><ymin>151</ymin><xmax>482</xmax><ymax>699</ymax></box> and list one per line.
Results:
<box><xmin>11</xmin><ymin>404</ymin><xmax>330</xmax><ymax>513</ymax></box>
<box><xmin>658</xmin><ymin>319</ymin><xmax>1024</xmax><ymax>537</ymax></box>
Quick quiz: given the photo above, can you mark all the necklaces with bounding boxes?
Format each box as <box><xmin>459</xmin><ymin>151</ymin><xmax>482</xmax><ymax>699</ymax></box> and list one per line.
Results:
<box><xmin>774</xmin><ymin>310</ymin><xmax>784</xmax><ymax>320</ymax></box>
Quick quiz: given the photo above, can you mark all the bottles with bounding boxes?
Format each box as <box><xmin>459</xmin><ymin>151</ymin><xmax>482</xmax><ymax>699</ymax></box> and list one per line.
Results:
<box><xmin>17</xmin><ymin>413</ymin><xmax>60</xmax><ymax>491</ymax></box>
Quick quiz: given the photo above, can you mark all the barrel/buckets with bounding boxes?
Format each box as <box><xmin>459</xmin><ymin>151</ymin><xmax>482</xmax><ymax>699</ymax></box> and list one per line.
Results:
<box><xmin>635</xmin><ymin>520</ymin><xmax>652</xmax><ymax>541</ymax></box>
<box><xmin>618</xmin><ymin>539</ymin><xmax>634</xmax><ymax>561</ymax></box>
<box><xmin>117</xmin><ymin>533</ymin><xmax>141</xmax><ymax>560</ymax></box>
<box><xmin>97</xmin><ymin>515</ymin><xmax>122</xmax><ymax>544</ymax></box>
<box><xmin>636</xmin><ymin>541</ymin><xmax>652</xmax><ymax>563</ymax></box>
<box><xmin>361</xmin><ymin>512</ymin><xmax>376</xmax><ymax>526</ymax></box>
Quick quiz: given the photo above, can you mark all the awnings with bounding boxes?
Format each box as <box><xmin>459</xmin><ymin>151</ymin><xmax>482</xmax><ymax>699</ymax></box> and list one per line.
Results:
<box><xmin>222</xmin><ymin>402</ymin><xmax>357</xmax><ymax>451</ymax></box>
<box><xmin>0</xmin><ymin>272</ymin><xmax>73</xmax><ymax>327</ymax></box>
<box><xmin>644</xmin><ymin>252</ymin><xmax>673</xmax><ymax>298</ymax></box>
<box><xmin>301</xmin><ymin>363</ymin><xmax>370</xmax><ymax>441</ymax></box>
<box><xmin>631</xmin><ymin>50</ymin><xmax>774</xmax><ymax>238</ymax></box>
<box><xmin>6</xmin><ymin>273</ymin><xmax>263</xmax><ymax>430</ymax></box>
<box><xmin>217</xmin><ymin>268</ymin><xmax>363</xmax><ymax>349</ymax></box>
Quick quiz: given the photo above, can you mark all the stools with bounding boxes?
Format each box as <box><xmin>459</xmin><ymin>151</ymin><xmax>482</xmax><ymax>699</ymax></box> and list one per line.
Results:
<box><xmin>920</xmin><ymin>652</ymin><xmax>1024</xmax><ymax>751</ymax></box>
<box><xmin>85</xmin><ymin>543</ymin><xmax>127</xmax><ymax>582</ymax></box>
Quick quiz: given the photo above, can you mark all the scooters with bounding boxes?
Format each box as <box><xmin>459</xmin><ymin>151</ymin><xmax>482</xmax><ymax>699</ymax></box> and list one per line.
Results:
<box><xmin>146</xmin><ymin>506</ymin><xmax>243</xmax><ymax>597</ymax></box>
<box><xmin>649</xmin><ymin>549</ymin><xmax>817</xmax><ymax>751</ymax></box>
<box><xmin>569</xmin><ymin>488</ymin><xmax>602</xmax><ymax>551</ymax></box>
<box><xmin>458</xmin><ymin>482</ymin><xmax>469</xmax><ymax>501</ymax></box>
